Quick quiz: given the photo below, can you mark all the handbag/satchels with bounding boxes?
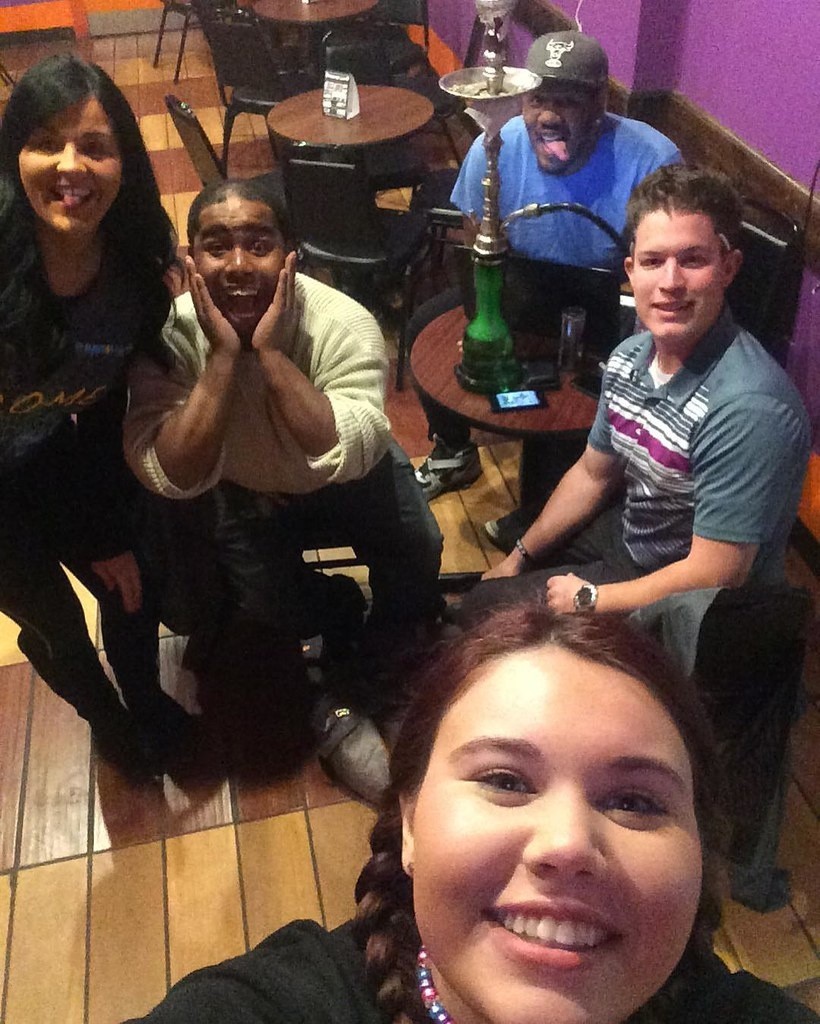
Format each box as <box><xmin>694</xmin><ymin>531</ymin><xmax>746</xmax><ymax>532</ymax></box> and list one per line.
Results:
<box><xmin>312</xmin><ymin>689</ymin><xmax>391</xmax><ymax>805</ymax></box>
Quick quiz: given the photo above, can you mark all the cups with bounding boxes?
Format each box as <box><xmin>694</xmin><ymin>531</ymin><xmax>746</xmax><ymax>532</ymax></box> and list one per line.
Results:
<box><xmin>554</xmin><ymin>306</ymin><xmax>586</xmax><ymax>373</ymax></box>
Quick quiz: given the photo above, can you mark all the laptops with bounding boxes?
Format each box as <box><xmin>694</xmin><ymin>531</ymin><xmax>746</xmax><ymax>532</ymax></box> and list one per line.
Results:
<box><xmin>453</xmin><ymin>244</ymin><xmax>637</xmax><ymax>360</ymax></box>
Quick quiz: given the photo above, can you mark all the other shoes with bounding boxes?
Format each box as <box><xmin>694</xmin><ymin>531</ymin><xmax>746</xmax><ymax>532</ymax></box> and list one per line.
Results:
<box><xmin>121</xmin><ymin>688</ymin><xmax>201</xmax><ymax>750</ymax></box>
<box><xmin>92</xmin><ymin>714</ymin><xmax>179</xmax><ymax>772</ymax></box>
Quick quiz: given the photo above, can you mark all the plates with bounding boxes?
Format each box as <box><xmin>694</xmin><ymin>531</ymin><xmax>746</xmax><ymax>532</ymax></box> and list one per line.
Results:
<box><xmin>435</xmin><ymin>66</ymin><xmax>544</xmax><ymax>98</ymax></box>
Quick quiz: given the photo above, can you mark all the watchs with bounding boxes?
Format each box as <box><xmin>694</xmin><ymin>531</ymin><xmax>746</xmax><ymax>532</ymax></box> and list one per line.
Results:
<box><xmin>573</xmin><ymin>584</ymin><xmax>598</xmax><ymax>614</ymax></box>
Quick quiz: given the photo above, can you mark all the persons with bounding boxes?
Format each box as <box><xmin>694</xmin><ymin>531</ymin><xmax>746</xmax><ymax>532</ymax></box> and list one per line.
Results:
<box><xmin>408</xmin><ymin>32</ymin><xmax>686</xmax><ymax>556</ymax></box>
<box><xmin>120</xmin><ymin>185</ymin><xmax>446</xmax><ymax>796</ymax></box>
<box><xmin>118</xmin><ymin>600</ymin><xmax>820</xmax><ymax>1024</ymax></box>
<box><xmin>463</xmin><ymin>165</ymin><xmax>811</xmax><ymax>910</ymax></box>
<box><xmin>0</xmin><ymin>49</ymin><xmax>224</xmax><ymax>785</ymax></box>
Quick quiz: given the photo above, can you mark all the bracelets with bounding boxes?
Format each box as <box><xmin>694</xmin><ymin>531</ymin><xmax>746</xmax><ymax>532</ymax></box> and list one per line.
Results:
<box><xmin>516</xmin><ymin>539</ymin><xmax>529</xmax><ymax>559</ymax></box>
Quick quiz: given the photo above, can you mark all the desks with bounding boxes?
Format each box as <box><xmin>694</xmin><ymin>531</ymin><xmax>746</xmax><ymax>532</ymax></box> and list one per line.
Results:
<box><xmin>409</xmin><ymin>300</ymin><xmax>608</xmax><ymax>569</ymax></box>
<box><xmin>249</xmin><ymin>0</ymin><xmax>379</xmax><ymax>55</ymax></box>
<box><xmin>267</xmin><ymin>83</ymin><xmax>433</xmax><ymax>170</ymax></box>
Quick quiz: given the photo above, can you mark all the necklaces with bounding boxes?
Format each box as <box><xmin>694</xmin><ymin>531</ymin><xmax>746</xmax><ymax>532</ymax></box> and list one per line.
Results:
<box><xmin>414</xmin><ymin>946</ymin><xmax>456</xmax><ymax>1024</ymax></box>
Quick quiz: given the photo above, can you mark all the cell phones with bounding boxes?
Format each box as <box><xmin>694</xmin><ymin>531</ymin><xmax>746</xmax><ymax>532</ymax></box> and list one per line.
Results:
<box><xmin>488</xmin><ymin>388</ymin><xmax>549</xmax><ymax>413</ymax></box>
<box><xmin>570</xmin><ymin>373</ymin><xmax>602</xmax><ymax>400</ymax></box>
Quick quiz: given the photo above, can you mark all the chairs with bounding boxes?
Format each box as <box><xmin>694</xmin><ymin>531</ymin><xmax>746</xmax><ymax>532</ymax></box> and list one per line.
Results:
<box><xmin>153</xmin><ymin>0</ymin><xmax>469</xmax><ymax>394</ymax></box>
<box><xmin>722</xmin><ymin>193</ymin><xmax>804</xmax><ymax>369</ymax></box>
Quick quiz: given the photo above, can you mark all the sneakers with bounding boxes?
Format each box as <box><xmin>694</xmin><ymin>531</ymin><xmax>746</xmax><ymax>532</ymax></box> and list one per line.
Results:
<box><xmin>414</xmin><ymin>433</ymin><xmax>482</xmax><ymax>502</ymax></box>
<box><xmin>485</xmin><ymin>508</ymin><xmax>533</xmax><ymax>546</ymax></box>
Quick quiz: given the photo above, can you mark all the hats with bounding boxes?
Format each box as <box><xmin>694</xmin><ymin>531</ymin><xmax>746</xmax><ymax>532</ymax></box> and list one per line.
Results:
<box><xmin>525</xmin><ymin>31</ymin><xmax>609</xmax><ymax>85</ymax></box>
